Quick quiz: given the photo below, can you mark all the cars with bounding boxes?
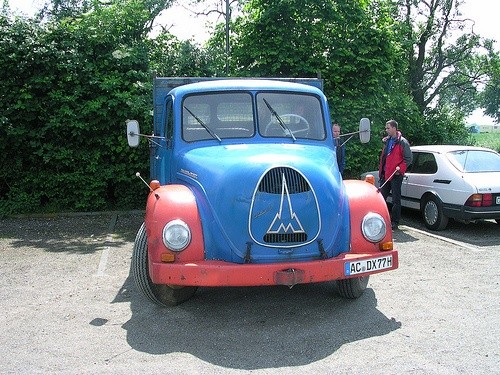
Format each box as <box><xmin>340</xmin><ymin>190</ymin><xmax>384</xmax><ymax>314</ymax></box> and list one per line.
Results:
<box><xmin>359</xmin><ymin>144</ymin><xmax>500</xmax><ymax>231</ymax></box>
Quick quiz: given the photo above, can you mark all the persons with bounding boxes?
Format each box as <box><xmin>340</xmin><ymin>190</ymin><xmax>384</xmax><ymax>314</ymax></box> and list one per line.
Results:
<box><xmin>332</xmin><ymin>124</ymin><xmax>345</xmax><ymax>177</ymax></box>
<box><xmin>378</xmin><ymin>120</ymin><xmax>413</xmax><ymax>229</ymax></box>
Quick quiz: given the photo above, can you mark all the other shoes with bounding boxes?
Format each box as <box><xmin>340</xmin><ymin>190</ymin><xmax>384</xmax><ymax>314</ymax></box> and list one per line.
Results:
<box><xmin>391</xmin><ymin>225</ymin><xmax>398</xmax><ymax>233</ymax></box>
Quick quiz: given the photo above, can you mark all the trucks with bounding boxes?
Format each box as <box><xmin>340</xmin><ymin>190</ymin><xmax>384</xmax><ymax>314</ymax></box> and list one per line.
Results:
<box><xmin>125</xmin><ymin>69</ymin><xmax>401</xmax><ymax>307</ymax></box>
